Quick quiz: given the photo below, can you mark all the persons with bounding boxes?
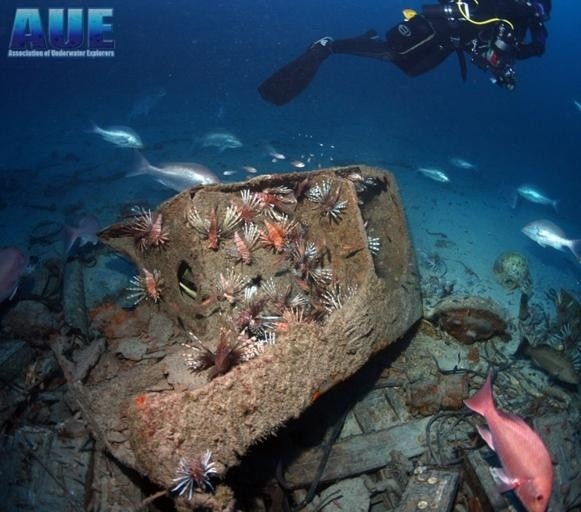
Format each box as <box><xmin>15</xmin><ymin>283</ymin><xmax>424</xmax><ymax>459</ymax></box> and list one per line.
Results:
<box><xmin>258</xmin><ymin>0</ymin><xmax>551</xmax><ymax>105</ymax></box>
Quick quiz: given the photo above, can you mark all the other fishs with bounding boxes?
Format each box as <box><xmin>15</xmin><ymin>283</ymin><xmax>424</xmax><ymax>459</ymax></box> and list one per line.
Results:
<box><xmin>185</xmin><ymin>131</ymin><xmax>382</xmax><ymax>383</ymax></box>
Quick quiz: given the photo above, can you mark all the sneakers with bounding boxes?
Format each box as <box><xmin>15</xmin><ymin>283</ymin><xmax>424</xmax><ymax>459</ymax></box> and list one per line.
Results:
<box><xmin>308</xmin><ymin>37</ymin><xmax>333</xmax><ymax>65</ymax></box>
